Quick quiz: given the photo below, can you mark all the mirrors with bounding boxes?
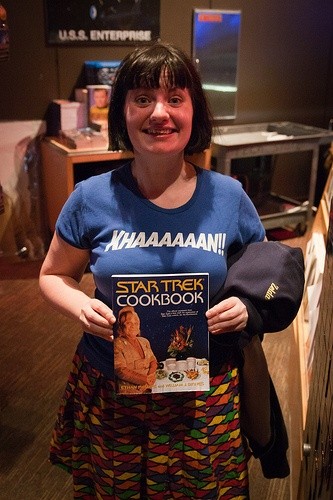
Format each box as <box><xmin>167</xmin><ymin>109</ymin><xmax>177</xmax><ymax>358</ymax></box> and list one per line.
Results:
<box><xmin>188</xmin><ymin>7</ymin><xmax>243</xmax><ymax>123</ymax></box>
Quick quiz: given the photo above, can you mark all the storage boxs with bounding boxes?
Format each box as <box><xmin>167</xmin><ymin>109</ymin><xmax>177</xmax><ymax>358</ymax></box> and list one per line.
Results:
<box><xmin>47</xmin><ymin>61</ymin><xmax>124</xmax><ymax>138</ymax></box>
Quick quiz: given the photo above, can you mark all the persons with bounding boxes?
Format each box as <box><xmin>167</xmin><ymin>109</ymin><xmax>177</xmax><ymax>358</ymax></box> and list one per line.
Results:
<box><xmin>114</xmin><ymin>310</ymin><xmax>158</xmax><ymax>395</ymax></box>
<box><xmin>37</xmin><ymin>39</ymin><xmax>272</xmax><ymax>500</ymax></box>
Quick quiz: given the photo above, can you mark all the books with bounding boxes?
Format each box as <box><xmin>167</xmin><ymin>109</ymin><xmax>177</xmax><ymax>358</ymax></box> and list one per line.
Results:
<box><xmin>49</xmin><ymin>84</ymin><xmax>111</xmax><ymax>152</ymax></box>
<box><xmin>110</xmin><ymin>271</ymin><xmax>211</xmax><ymax>395</ymax></box>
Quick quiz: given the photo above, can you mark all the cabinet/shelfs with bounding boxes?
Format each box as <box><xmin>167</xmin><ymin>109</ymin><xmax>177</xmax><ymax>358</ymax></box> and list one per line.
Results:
<box><xmin>37</xmin><ymin>137</ymin><xmax>213</xmax><ymax>237</ymax></box>
<box><xmin>203</xmin><ymin>121</ymin><xmax>333</xmax><ymax>244</ymax></box>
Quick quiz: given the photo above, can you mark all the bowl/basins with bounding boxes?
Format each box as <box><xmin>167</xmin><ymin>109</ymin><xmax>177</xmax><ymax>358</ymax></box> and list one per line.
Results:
<box><xmin>165</xmin><ymin>357</ymin><xmax>196</xmax><ymax>371</ymax></box>
